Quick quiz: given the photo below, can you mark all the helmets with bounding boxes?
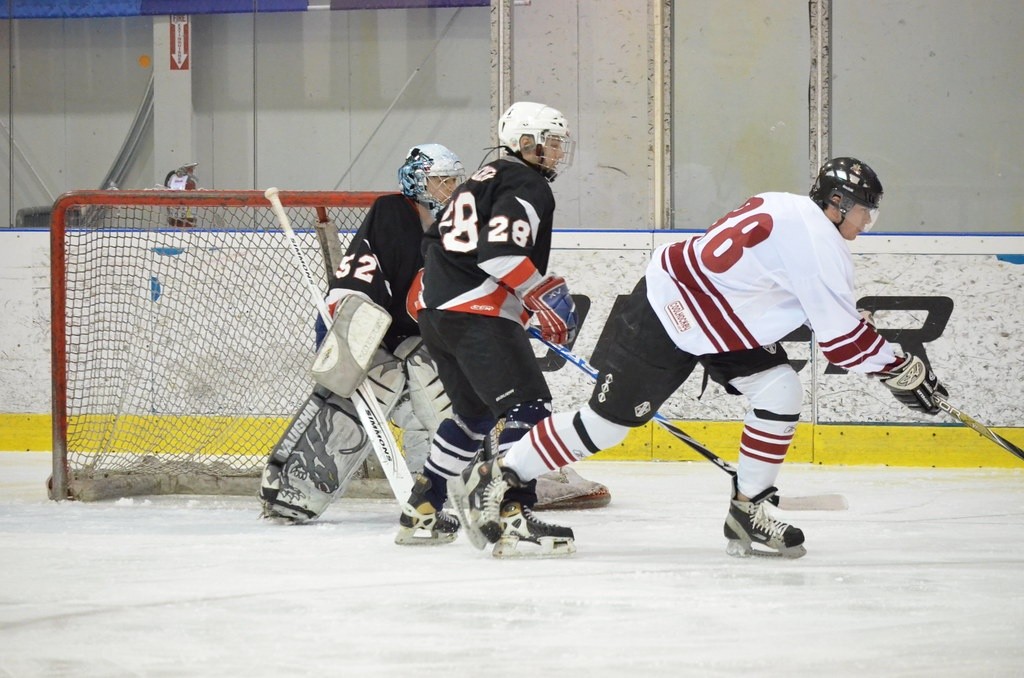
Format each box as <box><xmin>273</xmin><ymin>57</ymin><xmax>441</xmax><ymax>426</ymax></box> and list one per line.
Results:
<box><xmin>498</xmin><ymin>101</ymin><xmax>575</xmax><ymax>183</ymax></box>
<box><xmin>396</xmin><ymin>143</ymin><xmax>466</xmax><ymax>217</ymax></box>
<box><xmin>809</xmin><ymin>157</ymin><xmax>884</xmax><ymax>234</ymax></box>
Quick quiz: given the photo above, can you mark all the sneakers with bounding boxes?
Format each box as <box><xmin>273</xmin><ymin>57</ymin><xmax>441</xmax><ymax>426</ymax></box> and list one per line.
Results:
<box><xmin>722</xmin><ymin>473</ymin><xmax>807</xmax><ymax>558</ymax></box>
<box><xmin>445</xmin><ymin>452</ymin><xmax>513</xmax><ymax>550</ymax></box>
<box><xmin>393</xmin><ymin>474</ymin><xmax>460</xmax><ymax>545</ymax></box>
<box><xmin>491</xmin><ymin>497</ymin><xmax>576</xmax><ymax>561</ymax></box>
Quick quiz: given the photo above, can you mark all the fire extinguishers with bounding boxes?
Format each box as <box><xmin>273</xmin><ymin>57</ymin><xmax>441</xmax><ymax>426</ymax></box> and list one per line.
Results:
<box><xmin>165</xmin><ymin>162</ymin><xmax>200</xmax><ymax>227</ymax></box>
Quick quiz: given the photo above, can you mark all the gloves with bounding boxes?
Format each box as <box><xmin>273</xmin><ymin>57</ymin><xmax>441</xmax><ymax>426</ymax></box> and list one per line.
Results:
<box><xmin>520</xmin><ymin>273</ymin><xmax>580</xmax><ymax>344</ymax></box>
<box><xmin>876</xmin><ymin>351</ymin><xmax>950</xmax><ymax>415</ymax></box>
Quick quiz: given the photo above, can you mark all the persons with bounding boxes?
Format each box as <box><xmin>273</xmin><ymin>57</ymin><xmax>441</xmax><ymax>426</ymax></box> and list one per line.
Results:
<box><xmin>446</xmin><ymin>157</ymin><xmax>949</xmax><ymax>560</ymax></box>
<box><xmin>258</xmin><ymin>101</ymin><xmax>611</xmax><ymax>558</ymax></box>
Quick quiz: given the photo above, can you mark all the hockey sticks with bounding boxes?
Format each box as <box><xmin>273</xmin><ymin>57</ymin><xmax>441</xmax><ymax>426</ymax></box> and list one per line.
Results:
<box><xmin>264</xmin><ymin>184</ymin><xmax>416</xmax><ymax>509</ymax></box>
<box><xmin>525</xmin><ymin>322</ymin><xmax>853</xmax><ymax>512</ymax></box>
<box><xmin>932</xmin><ymin>394</ymin><xmax>1024</xmax><ymax>461</ymax></box>
